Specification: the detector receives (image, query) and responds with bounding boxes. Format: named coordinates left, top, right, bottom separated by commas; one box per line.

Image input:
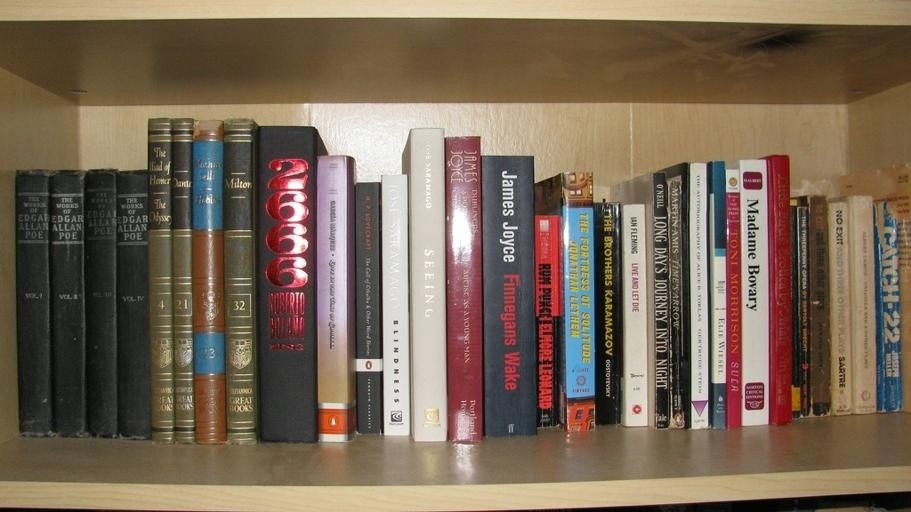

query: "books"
left=16, top=118, right=911, bottom=447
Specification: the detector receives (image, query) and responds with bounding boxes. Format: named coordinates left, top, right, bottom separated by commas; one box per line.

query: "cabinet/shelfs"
left=1, top=1, right=911, bottom=511
